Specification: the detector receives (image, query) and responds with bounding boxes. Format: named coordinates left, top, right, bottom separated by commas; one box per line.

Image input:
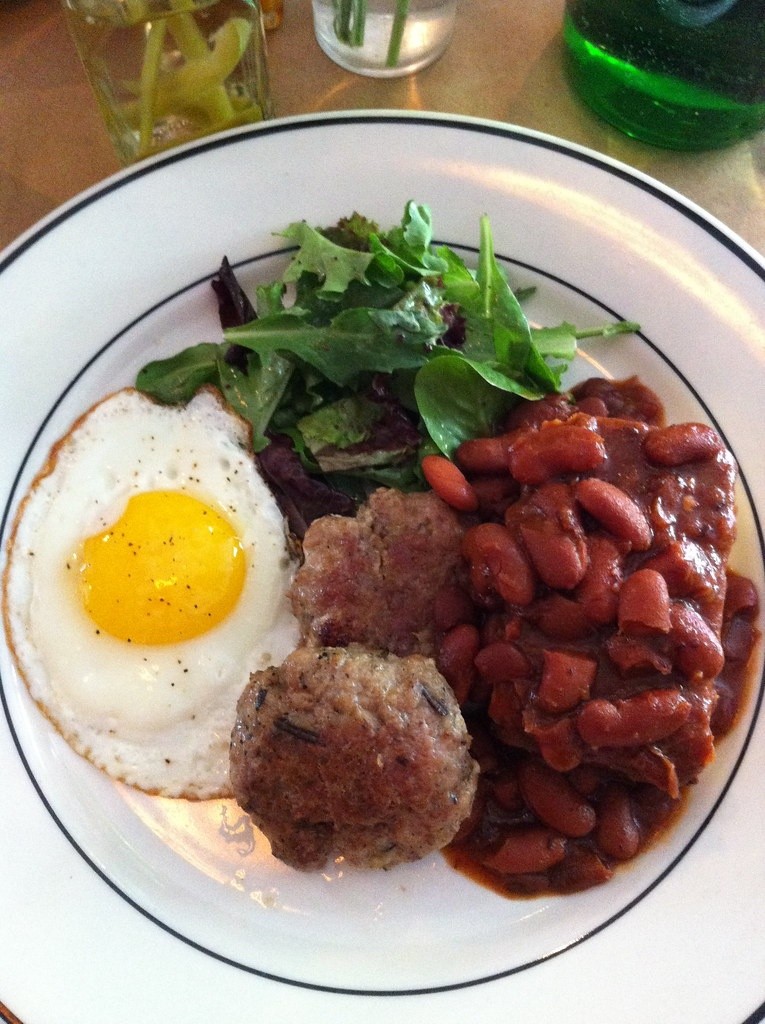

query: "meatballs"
left=226, top=484, right=487, bottom=877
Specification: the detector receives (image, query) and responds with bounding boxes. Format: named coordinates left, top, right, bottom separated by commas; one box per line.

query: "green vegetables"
left=123, top=197, right=646, bottom=536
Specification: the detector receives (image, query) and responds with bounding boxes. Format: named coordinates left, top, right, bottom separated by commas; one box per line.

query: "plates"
left=0, top=108, right=761, bottom=1024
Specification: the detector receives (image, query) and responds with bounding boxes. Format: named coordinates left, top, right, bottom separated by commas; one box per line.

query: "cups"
left=255, top=0, right=285, bottom=33
left=60, top=0, right=273, bottom=172
left=312, top=0, right=459, bottom=79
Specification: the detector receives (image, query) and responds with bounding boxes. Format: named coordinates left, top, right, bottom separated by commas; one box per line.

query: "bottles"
left=558, top=1, right=764, bottom=157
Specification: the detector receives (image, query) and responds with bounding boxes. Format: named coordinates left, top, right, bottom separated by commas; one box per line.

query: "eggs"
left=3, top=383, right=299, bottom=805
